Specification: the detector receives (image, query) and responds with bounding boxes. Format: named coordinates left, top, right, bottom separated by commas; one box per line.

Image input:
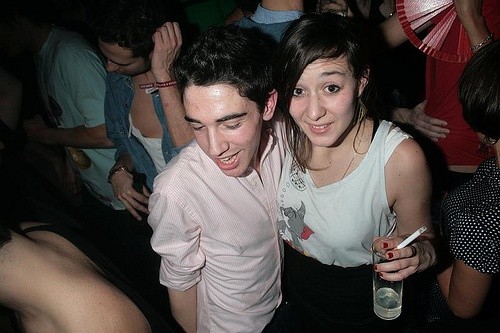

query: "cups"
left=371, top=237, right=404, bottom=320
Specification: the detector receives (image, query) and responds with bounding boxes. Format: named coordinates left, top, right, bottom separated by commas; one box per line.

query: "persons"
left=0, top=0, right=499, bottom=333
